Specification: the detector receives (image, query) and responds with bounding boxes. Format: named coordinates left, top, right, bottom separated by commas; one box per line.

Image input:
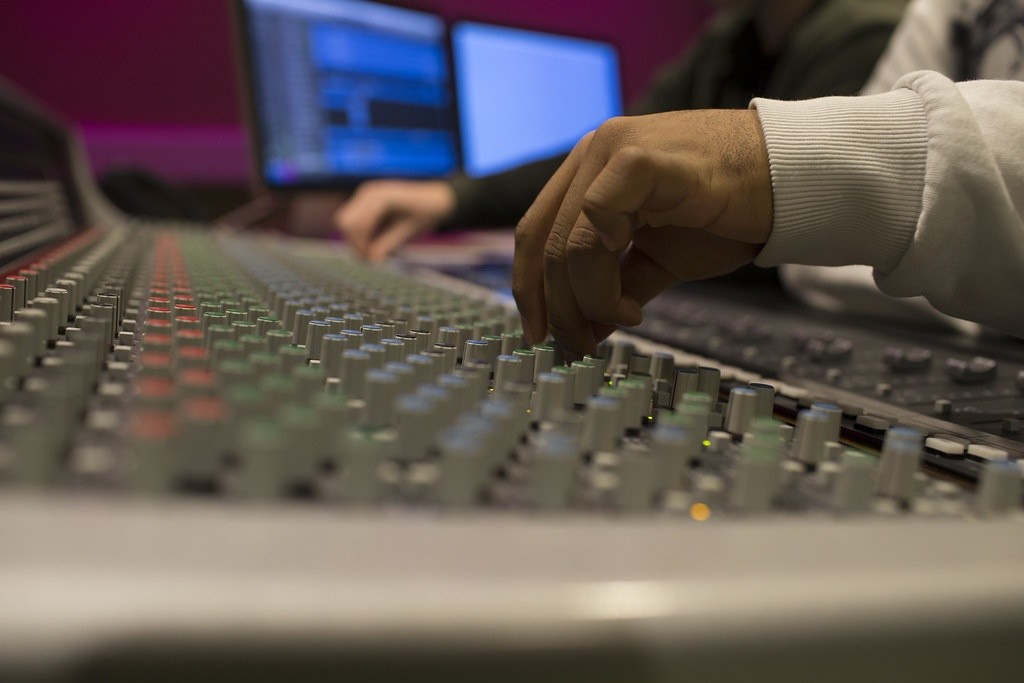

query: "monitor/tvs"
left=445, top=19, right=625, bottom=182
left=229, top=0, right=454, bottom=195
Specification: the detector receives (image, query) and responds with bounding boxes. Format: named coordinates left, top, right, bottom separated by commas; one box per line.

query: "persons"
left=513, top=0, right=1024, bottom=367
left=333, top=0, right=905, bottom=263
